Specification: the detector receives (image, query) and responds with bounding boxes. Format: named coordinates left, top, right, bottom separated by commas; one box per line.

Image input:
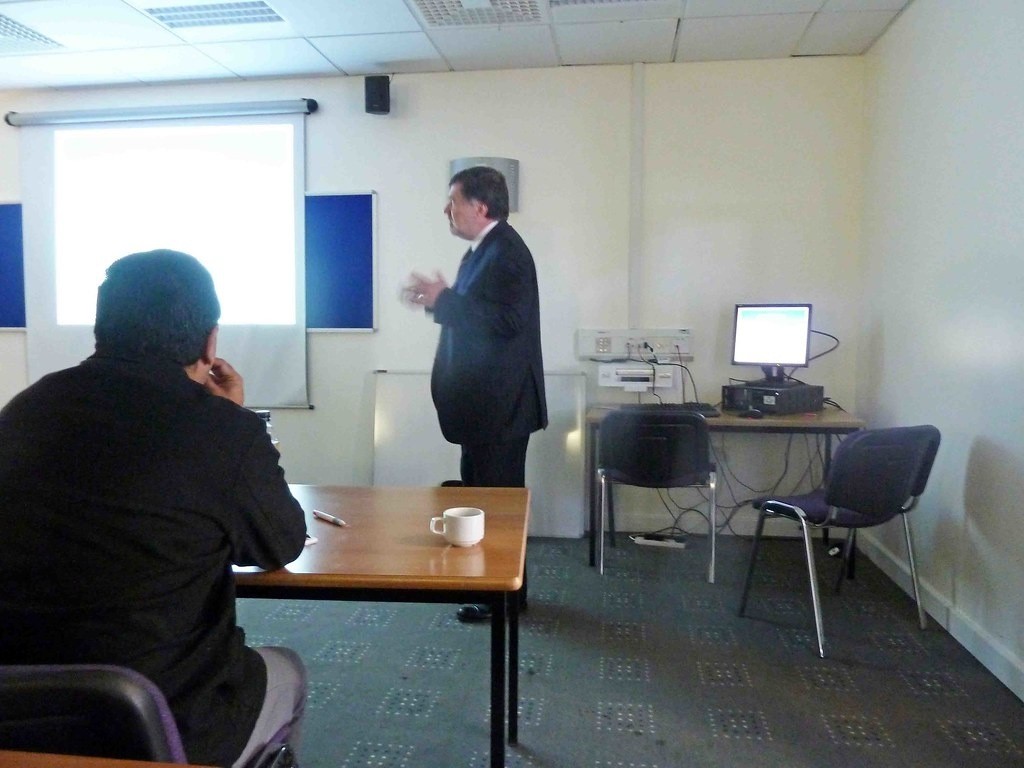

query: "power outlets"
left=627, top=326, right=695, bottom=359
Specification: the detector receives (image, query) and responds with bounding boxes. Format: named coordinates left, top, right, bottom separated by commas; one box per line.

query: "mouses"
left=739, top=409, right=761, bottom=419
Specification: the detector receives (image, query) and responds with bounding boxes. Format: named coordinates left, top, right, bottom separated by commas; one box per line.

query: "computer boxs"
left=721, top=384, right=825, bottom=417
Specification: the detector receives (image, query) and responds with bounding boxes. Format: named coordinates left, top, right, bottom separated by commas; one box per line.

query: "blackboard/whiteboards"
left=0, top=191, right=380, bottom=334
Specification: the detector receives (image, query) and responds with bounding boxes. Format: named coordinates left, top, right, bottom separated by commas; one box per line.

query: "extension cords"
left=635, top=535, right=686, bottom=549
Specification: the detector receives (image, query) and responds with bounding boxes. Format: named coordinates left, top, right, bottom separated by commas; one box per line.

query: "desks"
left=586, top=402, right=866, bottom=580
left=233, top=483, right=532, bottom=768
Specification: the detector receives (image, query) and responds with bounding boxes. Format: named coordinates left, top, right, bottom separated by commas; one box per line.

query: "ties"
left=457, top=246, right=473, bottom=279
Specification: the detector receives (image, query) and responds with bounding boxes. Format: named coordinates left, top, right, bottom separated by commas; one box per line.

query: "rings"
left=416, top=293, right=422, bottom=299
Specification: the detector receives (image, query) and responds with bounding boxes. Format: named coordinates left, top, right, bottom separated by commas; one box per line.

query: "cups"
left=429, top=507, right=486, bottom=547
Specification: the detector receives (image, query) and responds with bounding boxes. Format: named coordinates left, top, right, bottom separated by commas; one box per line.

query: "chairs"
left=0, top=664, right=294, bottom=768
left=595, top=411, right=718, bottom=585
left=737, top=423, right=942, bottom=659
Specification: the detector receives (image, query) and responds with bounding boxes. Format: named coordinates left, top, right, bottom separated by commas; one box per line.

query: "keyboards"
left=620, top=403, right=721, bottom=418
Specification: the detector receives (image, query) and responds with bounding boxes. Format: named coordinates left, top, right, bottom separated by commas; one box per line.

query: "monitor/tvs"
left=732, top=303, right=812, bottom=386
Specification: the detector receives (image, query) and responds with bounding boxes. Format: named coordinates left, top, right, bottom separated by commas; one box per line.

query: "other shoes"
left=503, top=599, right=528, bottom=620
left=457, top=604, right=492, bottom=620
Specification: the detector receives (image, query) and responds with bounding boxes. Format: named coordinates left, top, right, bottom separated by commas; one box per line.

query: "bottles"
left=255, top=408, right=281, bottom=464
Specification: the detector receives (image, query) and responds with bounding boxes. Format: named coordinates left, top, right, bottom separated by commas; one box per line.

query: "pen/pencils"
left=312, top=509, right=346, bottom=527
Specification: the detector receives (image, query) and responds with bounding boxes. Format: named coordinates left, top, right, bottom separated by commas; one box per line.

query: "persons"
left=401, top=167, right=549, bottom=624
left=0, top=249, right=306, bottom=768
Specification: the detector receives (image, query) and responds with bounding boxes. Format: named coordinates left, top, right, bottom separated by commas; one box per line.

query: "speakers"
left=363, top=75, right=390, bottom=114
